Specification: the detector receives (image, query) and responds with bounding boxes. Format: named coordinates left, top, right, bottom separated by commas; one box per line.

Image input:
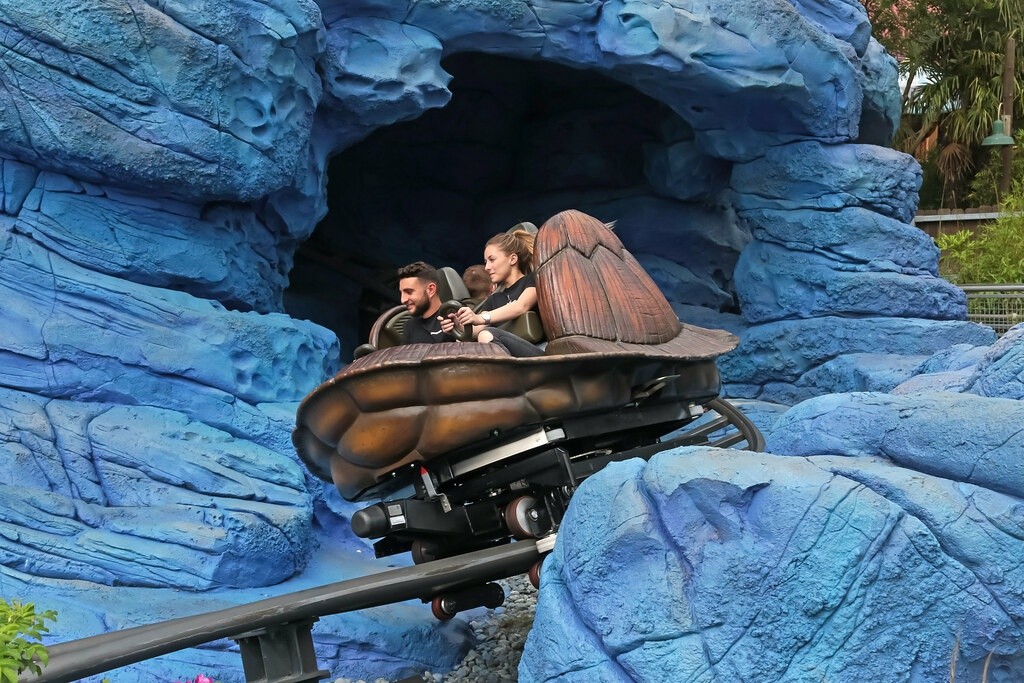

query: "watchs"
left=481, top=310, right=491, bottom=325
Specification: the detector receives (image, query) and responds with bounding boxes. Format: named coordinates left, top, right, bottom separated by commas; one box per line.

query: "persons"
left=396, top=231, right=537, bottom=343
